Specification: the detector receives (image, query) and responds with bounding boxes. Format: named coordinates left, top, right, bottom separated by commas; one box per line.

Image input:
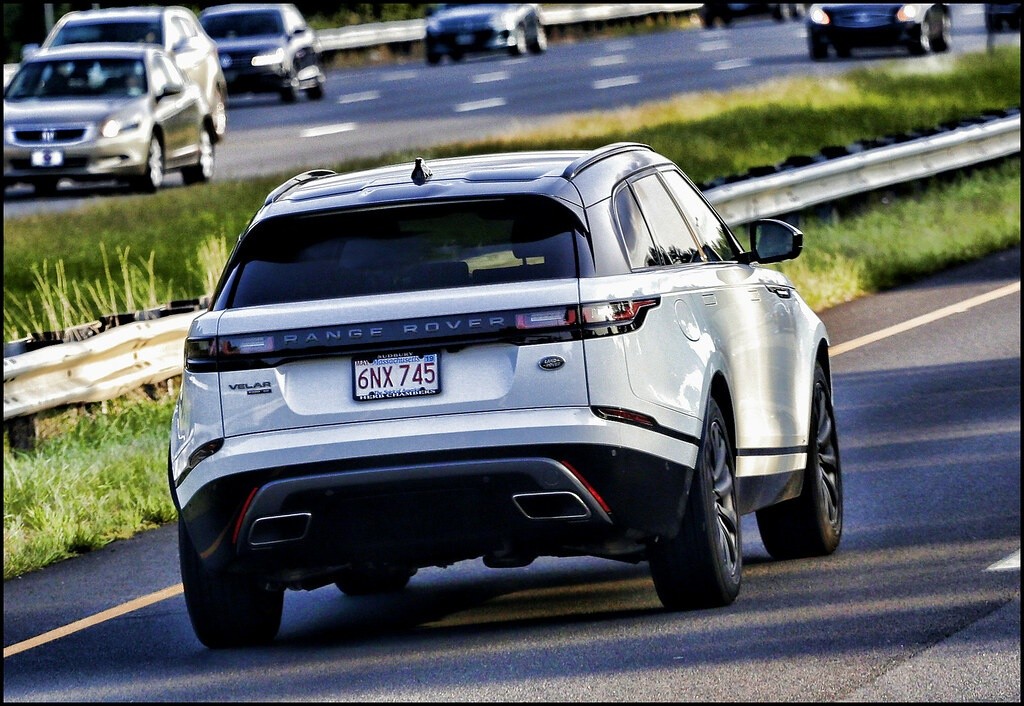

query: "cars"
left=806, top=3, right=954, bottom=57
left=985, top=4, right=1020, bottom=32
left=3, top=42, right=216, bottom=190
left=696, top=4, right=807, bottom=27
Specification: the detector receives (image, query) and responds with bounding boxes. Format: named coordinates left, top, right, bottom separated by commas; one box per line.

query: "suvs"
left=424, top=3, right=548, bottom=63
left=166, top=140, right=844, bottom=647
left=37, top=7, right=229, bottom=142
left=199, top=3, right=326, bottom=103
